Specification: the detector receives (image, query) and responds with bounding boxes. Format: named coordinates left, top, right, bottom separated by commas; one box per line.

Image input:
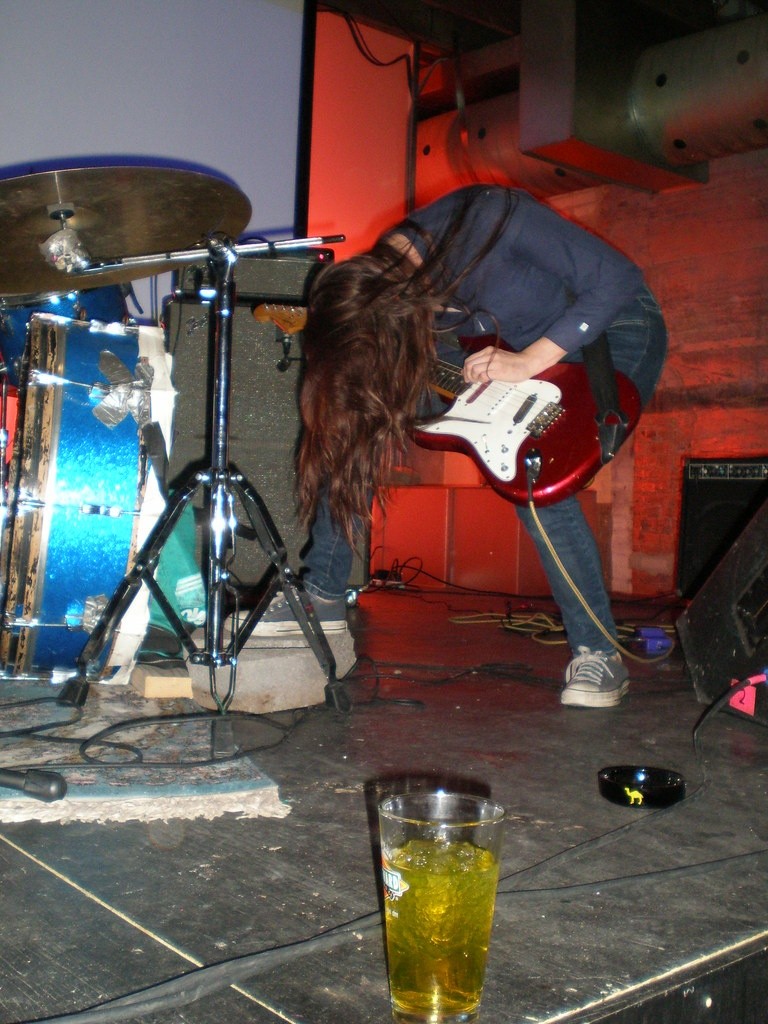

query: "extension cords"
left=371, top=579, right=402, bottom=588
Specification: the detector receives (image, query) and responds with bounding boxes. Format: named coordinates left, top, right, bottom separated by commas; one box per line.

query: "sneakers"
left=224, top=584, right=347, bottom=638
left=560, top=645, right=629, bottom=707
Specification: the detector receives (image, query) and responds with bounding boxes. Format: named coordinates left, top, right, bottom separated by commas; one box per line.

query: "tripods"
left=54, top=260, right=354, bottom=762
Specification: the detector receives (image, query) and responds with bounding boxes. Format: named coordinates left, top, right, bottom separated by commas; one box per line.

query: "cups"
left=376, top=791, right=506, bottom=1024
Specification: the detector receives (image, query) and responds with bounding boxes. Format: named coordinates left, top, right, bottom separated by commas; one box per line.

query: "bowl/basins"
left=598, top=764, right=685, bottom=809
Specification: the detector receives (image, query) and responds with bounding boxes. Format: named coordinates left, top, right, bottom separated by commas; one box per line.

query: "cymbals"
left=0, top=164, right=253, bottom=298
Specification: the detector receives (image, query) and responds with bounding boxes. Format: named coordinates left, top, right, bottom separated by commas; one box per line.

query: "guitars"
left=251, top=298, right=643, bottom=509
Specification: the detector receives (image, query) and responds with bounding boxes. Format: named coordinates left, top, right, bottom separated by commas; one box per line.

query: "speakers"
left=676, top=497, right=768, bottom=725
left=675, top=455, right=767, bottom=609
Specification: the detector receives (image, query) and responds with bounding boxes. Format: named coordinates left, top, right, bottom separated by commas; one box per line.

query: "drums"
left=1, top=311, right=155, bottom=680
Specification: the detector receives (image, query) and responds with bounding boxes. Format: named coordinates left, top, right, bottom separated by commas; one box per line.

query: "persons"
left=225, top=184, right=669, bottom=708
